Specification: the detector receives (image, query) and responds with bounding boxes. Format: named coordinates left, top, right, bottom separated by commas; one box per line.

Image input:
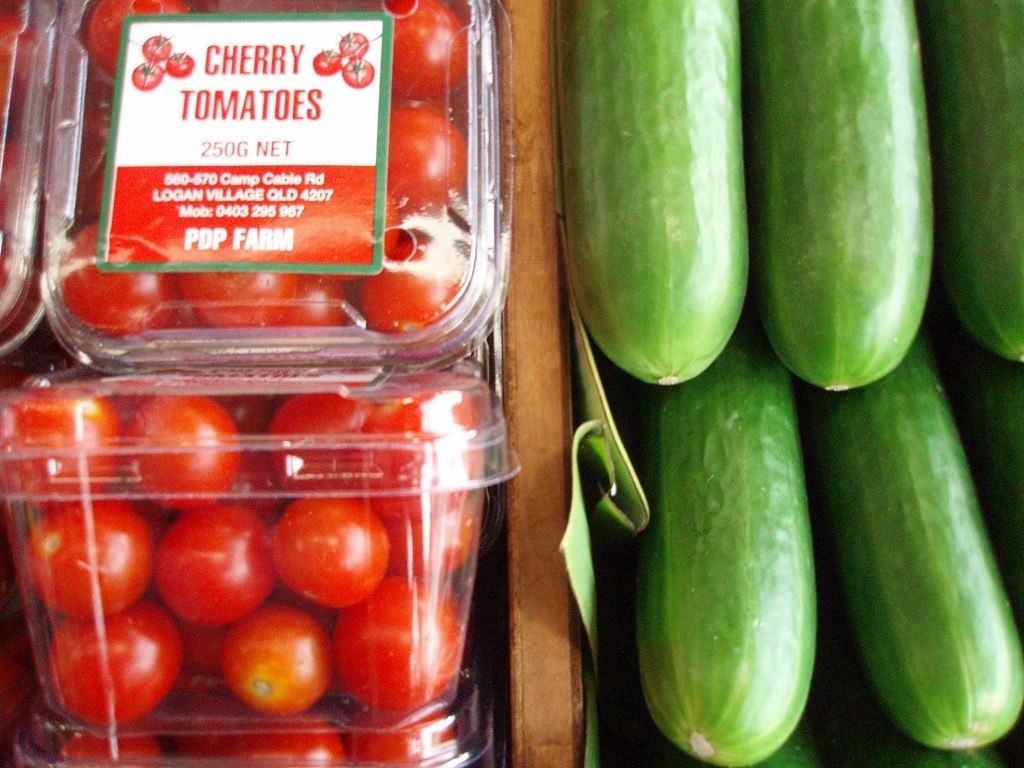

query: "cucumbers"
left=558, top=0, right=1024, bottom=768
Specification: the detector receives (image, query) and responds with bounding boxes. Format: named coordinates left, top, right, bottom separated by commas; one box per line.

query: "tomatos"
left=0, top=0, right=494, bottom=768
